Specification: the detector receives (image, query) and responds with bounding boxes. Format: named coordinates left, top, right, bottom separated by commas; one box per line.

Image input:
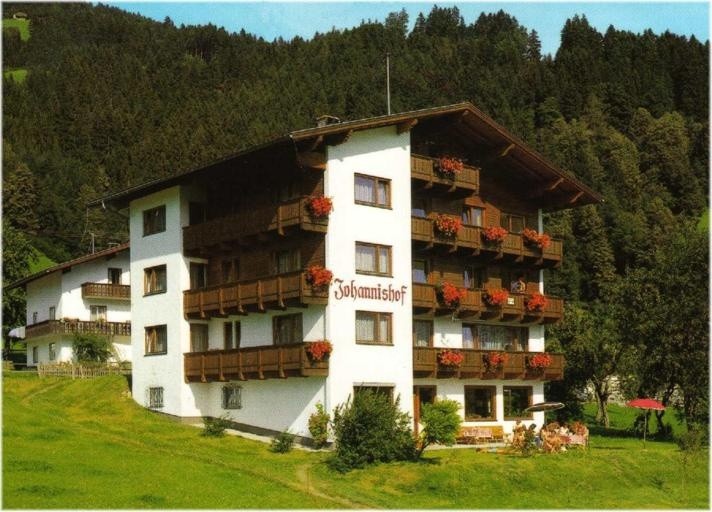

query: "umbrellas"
left=523, top=401, right=565, bottom=425
left=625, top=399, right=666, bottom=448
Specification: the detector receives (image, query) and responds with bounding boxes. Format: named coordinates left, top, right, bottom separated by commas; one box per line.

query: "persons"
left=476, top=448, right=513, bottom=454
left=512, top=277, right=525, bottom=293
left=512, top=419, right=587, bottom=453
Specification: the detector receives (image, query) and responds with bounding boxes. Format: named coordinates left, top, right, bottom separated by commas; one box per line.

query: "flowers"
left=433, top=158, right=553, bottom=375
left=304, top=197, right=332, bottom=367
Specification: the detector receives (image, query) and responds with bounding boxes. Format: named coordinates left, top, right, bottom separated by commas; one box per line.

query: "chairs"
left=456, top=418, right=591, bottom=453
